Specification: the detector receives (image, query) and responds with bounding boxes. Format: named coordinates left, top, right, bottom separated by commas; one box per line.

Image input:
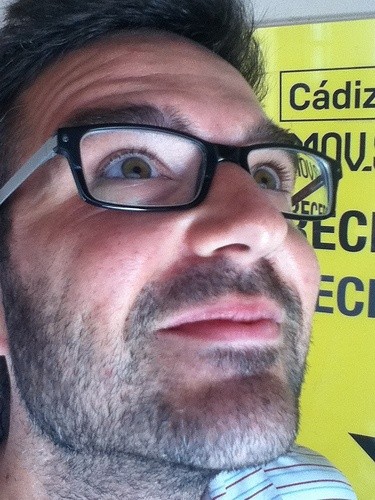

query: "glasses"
left=1, top=122, right=344, bottom=222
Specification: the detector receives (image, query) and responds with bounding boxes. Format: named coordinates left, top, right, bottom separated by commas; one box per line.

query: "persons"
left=0, top=0, right=360, bottom=500
